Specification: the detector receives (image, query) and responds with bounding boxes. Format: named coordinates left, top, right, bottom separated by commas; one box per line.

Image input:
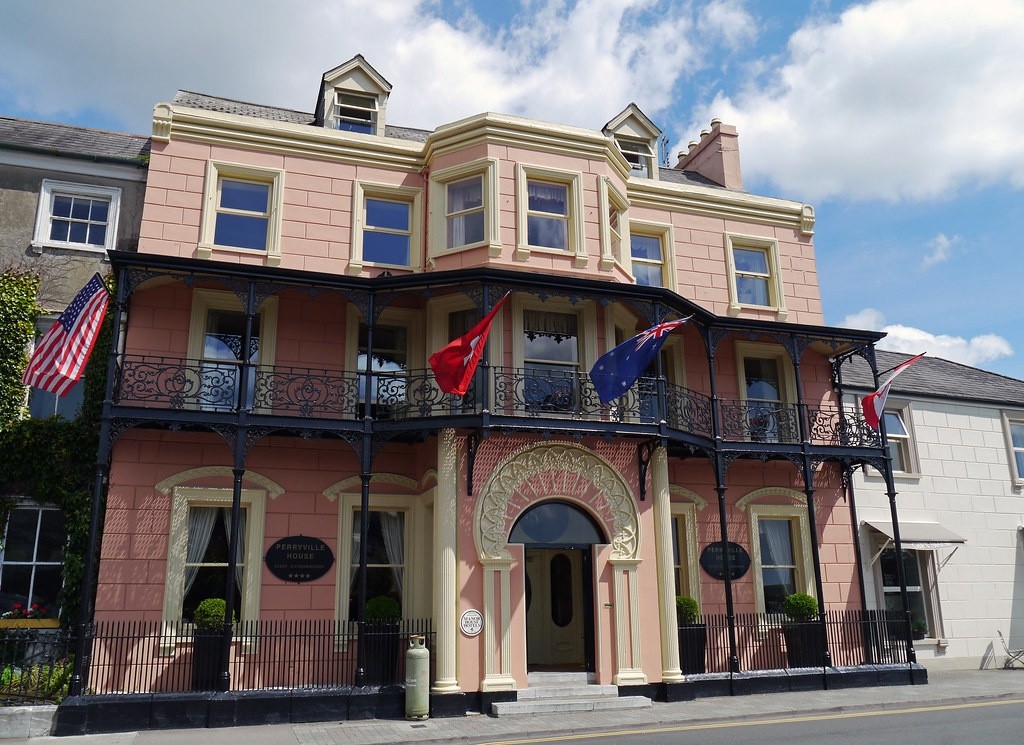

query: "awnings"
left=866, top=522, right=968, bottom=549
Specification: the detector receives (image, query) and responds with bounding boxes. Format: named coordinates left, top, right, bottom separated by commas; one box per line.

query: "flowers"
left=1, top=603, right=49, bottom=619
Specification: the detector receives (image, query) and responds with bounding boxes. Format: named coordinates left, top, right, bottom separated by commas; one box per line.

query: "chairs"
left=997, top=630, right=1024, bottom=669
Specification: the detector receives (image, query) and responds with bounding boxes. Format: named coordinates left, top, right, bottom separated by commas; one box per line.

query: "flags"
left=22, top=272, right=111, bottom=398
left=589, top=313, right=695, bottom=404
left=861, top=352, right=925, bottom=432
left=428, top=289, right=512, bottom=397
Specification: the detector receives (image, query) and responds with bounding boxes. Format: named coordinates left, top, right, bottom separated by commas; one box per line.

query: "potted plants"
left=675, top=597, right=707, bottom=675
left=192, top=598, right=236, bottom=692
left=912, top=619, right=927, bottom=639
left=364, top=597, right=401, bottom=685
left=782, top=592, right=825, bottom=668
